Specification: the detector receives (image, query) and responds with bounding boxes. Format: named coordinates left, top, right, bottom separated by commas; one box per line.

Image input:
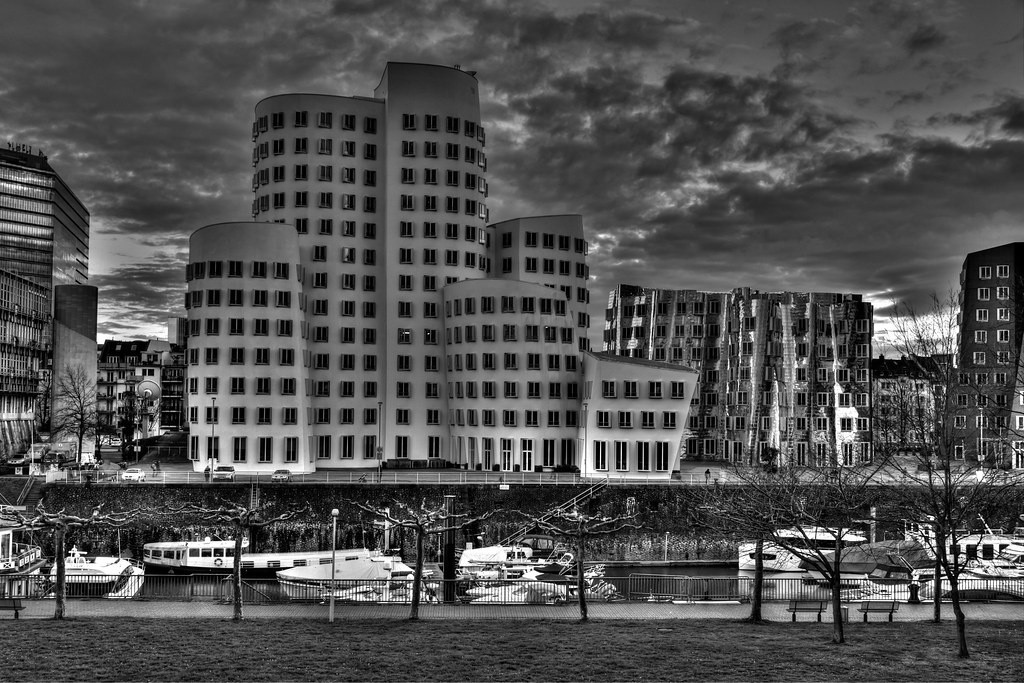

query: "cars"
left=271, top=469, right=294, bottom=482
left=7, top=453, right=32, bottom=467
left=121, top=468, right=146, bottom=482
left=27, top=442, right=99, bottom=472
left=111, top=438, right=122, bottom=447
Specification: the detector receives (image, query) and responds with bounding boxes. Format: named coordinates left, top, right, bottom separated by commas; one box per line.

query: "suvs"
left=213, top=465, right=236, bottom=483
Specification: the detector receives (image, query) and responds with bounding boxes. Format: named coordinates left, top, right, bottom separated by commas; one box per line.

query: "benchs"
left=0, top=598, right=27, bottom=620
left=857, top=601, right=900, bottom=623
left=787, top=599, right=827, bottom=622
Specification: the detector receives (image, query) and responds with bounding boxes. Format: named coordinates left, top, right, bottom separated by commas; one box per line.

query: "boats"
left=0, top=527, right=46, bottom=599
left=43, top=545, right=147, bottom=602
left=139, top=539, right=621, bottom=606
left=737, top=525, right=1023, bottom=604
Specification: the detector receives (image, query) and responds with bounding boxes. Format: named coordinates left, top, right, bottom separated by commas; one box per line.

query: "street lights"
left=583, top=402, right=591, bottom=482
left=377, top=401, right=383, bottom=483
left=211, top=396, right=215, bottom=482
left=328, top=508, right=340, bottom=622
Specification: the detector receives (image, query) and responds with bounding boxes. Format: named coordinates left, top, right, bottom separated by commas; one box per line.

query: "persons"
left=705, top=469, right=711, bottom=484
left=204, top=466, right=210, bottom=482
left=150, top=459, right=160, bottom=478
left=377, top=465, right=383, bottom=483
